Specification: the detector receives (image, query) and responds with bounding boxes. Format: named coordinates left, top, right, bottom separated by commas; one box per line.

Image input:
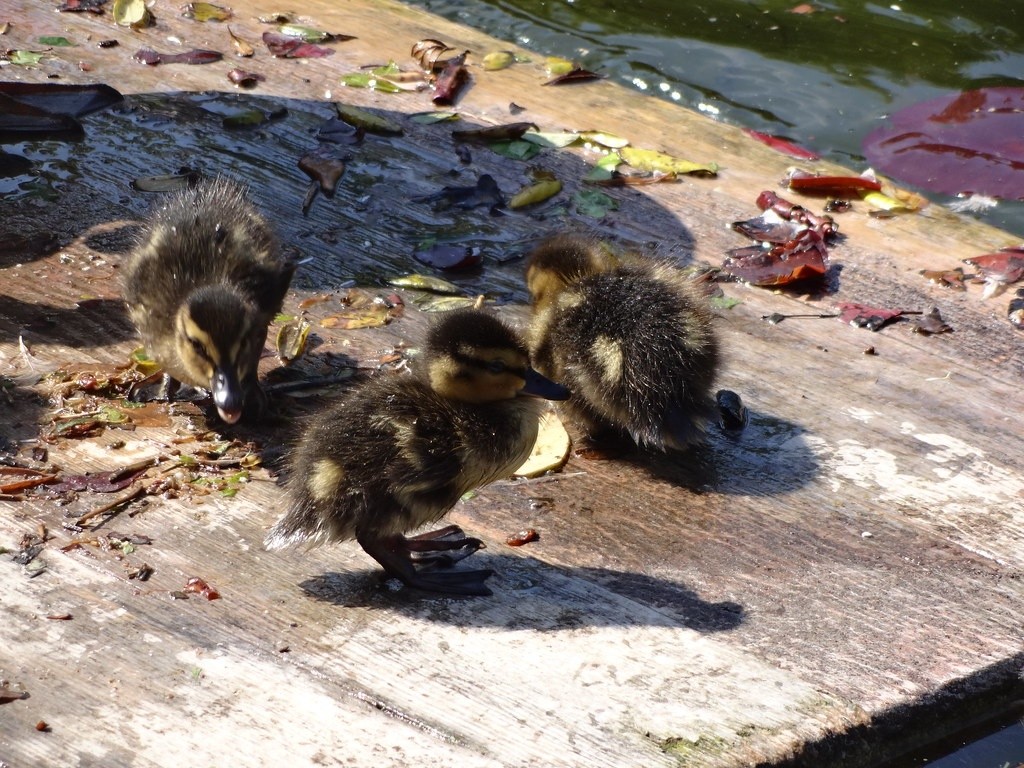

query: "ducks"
left=519, top=233, right=721, bottom=441
left=113, top=173, right=296, bottom=425
left=259, top=306, right=574, bottom=598
